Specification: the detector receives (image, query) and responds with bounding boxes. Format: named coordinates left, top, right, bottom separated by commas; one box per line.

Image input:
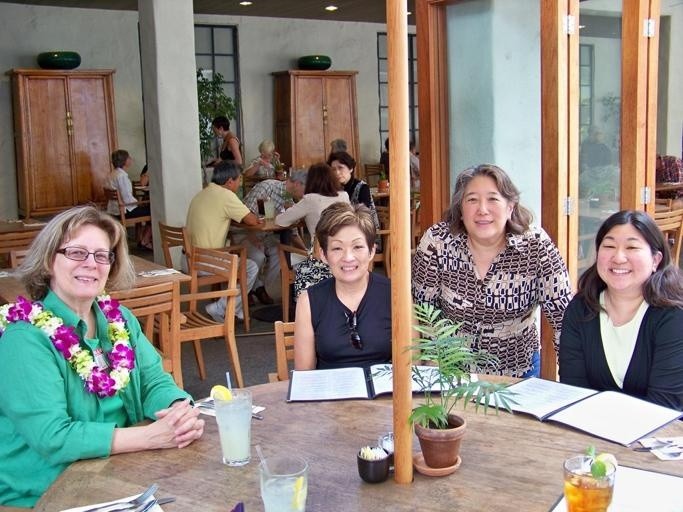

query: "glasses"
left=344, top=311, right=363, bottom=351
left=56, top=247, right=116, bottom=265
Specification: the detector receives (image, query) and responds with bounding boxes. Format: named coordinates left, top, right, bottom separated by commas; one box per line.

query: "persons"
left=293, top=202, right=421, bottom=372
left=1, top=206, right=205, bottom=507
left=139, top=162, right=150, bottom=186
left=231, top=168, right=308, bottom=305
left=581, top=127, right=611, bottom=176
left=411, top=163, right=572, bottom=378
left=243, top=139, right=279, bottom=179
left=327, top=151, right=384, bottom=253
left=330, top=140, right=347, bottom=152
left=106, top=150, right=153, bottom=250
left=381, top=138, right=421, bottom=196
left=560, top=209, right=683, bottom=412
left=208, top=116, right=242, bottom=166
left=275, top=163, right=354, bottom=302
left=184, top=160, right=259, bottom=323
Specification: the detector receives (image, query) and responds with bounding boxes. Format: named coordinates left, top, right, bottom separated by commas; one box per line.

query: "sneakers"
left=206, top=301, right=225, bottom=323
left=235, top=307, right=251, bottom=322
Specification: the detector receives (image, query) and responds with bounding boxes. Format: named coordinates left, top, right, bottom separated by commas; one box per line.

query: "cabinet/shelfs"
left=5, top=68, right=118, bottom=222
left=270, top=70, right=362, bottom=180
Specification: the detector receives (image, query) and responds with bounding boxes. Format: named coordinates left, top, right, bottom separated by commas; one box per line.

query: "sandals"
left=137, top=240, right=153, bottom=253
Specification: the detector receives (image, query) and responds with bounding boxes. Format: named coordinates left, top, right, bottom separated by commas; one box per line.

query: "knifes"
left=109, top=499, right=174, bottom=512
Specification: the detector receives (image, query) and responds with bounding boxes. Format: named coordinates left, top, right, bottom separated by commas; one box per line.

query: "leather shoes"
left=248, top=291, right=255, bottom=307
left=255, top=286, right=274, bottom=305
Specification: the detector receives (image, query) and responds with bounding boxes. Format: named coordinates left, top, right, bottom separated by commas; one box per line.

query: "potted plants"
left=370, top=301, right=524, bottom=468
left=357, top=445, right=391, bottom=484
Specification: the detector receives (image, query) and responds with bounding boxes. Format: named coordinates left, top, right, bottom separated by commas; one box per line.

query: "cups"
left=214, top=389, right=253, bottom=467
left=564, top=455, right=616, bottom=512
left=358, top=446, right=388, bottom=485
left=260, top=454, right=307, bottom=511
left=382, top=435, right=394, bottom=471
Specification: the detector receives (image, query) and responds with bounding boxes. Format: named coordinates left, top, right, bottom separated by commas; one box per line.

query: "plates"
left=413, top=451, right=460, bottom=477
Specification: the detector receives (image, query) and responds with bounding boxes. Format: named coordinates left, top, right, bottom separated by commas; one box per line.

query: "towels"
left=23, top=222, right=48, bottom=226
left=1, top=271, right=16, bottom=277
left=138, top=268, right=180, bottom=277
left=639, top=438, right=683, bottom=462
left=192, top=395, right=266, bottom=419
left=57, top=492, right=167, bottom=512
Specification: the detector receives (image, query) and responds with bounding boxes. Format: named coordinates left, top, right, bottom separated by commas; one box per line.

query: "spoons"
left=134, top=500, right=154, bottom=512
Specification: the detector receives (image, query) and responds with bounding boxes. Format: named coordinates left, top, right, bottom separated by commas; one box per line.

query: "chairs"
left=135, top=248, right=243, bottom=390
left=0, top=233, right=42, bottom=255
left=132, top=181, right=147, bottom=201
left=11, top=249, right=28, bottom=268
left=655, top=180, right=676, bottom=193
left=103, top=187, right=151, bottom=229
left=367, top=205, right=391, bottom=280
left=365, top=164, right=384, bottom=185
left=652, top=209, right=679, bottom=269
left=653, top=199, right=671, bottom=243
left=277, top=236, right=322, bottom=323
left=109, top=280, right=182, bottom=391
left=157, top=222, right=250, bottom=333
left=655, top=209, right=683, bottom=271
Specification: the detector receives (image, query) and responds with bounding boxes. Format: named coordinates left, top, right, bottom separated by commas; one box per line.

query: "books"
left=473, top=378, right=683, bottom=446
left=285, top=364, right=456, bottom=402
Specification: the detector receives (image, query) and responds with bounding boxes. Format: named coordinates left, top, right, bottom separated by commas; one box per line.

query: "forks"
left=198, top=401, right=264, bottom=421
left=83, top=483, right=158, bottom=512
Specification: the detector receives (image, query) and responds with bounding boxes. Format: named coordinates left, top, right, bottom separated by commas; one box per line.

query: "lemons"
left=590, top=454, right=616, bottom=477
left=292, top=476, right=305, bottom=512
left=209, top=384, right=232, bottom=404
